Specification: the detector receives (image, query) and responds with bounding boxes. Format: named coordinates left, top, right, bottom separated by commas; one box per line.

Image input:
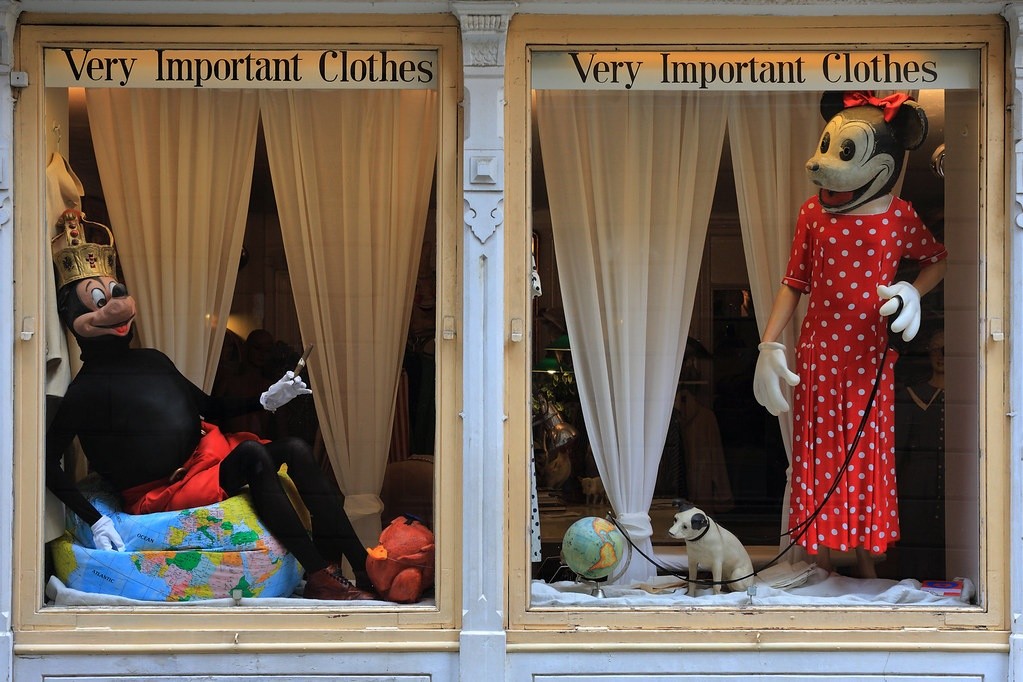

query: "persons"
left=753, top=89, right=949, bottom=578
left=44, top=208, right=386, bottom=602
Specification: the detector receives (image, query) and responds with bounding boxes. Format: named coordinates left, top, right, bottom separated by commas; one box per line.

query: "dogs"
left=668, top=499, right=754, bottom=597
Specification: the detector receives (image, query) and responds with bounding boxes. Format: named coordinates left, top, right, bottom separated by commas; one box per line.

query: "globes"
left=560, top=511, right=633, bottom=598
